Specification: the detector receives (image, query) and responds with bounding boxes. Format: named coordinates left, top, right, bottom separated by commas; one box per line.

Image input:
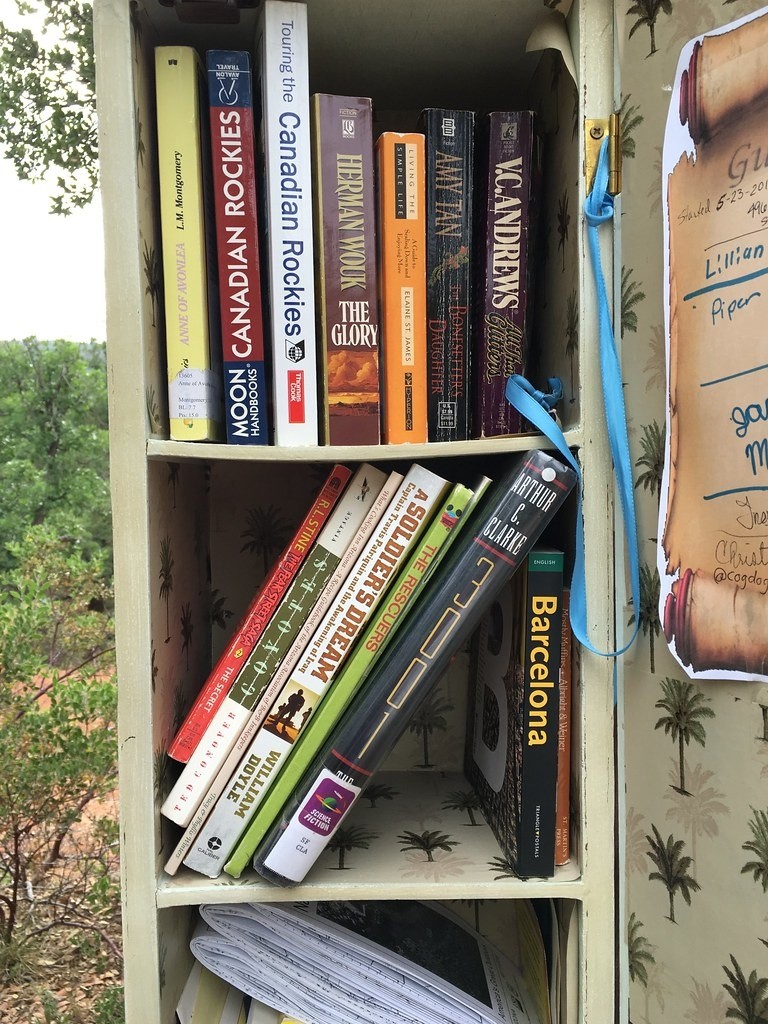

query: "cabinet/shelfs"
left=92, top=0, right=618, bottom=1024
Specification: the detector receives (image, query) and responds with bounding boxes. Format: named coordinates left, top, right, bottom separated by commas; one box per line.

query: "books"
left=153, top=0, right=533, bottom=447
left=158, top=448, right=579, bottom=890
